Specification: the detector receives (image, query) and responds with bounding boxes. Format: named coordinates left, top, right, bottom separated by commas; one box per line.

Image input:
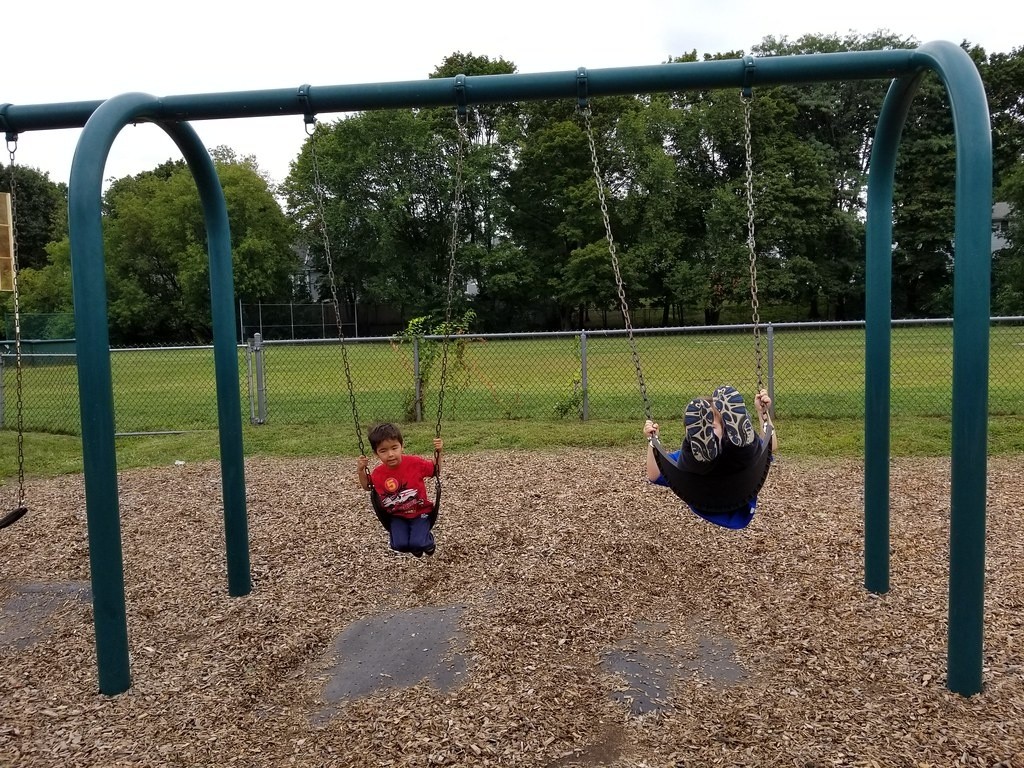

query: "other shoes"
left=424, top=532, right=435, bottom=556
left=712, top=385, right=754, bottom=447
left=684, top=399, right=718, bottom=462
left=411, top=551, right=423, bottom=557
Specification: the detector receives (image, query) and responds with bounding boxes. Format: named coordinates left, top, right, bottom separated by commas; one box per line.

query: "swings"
left=0, top=129, right=30, bottom=527
left=581, top=89, right=776, bottom=510
left=303, top=112, right=463, bottom=529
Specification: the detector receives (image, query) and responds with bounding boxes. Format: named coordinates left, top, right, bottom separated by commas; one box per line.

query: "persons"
left=643, top=384, right=777, bottom=529
left=356, top=422, right=443, bottom=558
